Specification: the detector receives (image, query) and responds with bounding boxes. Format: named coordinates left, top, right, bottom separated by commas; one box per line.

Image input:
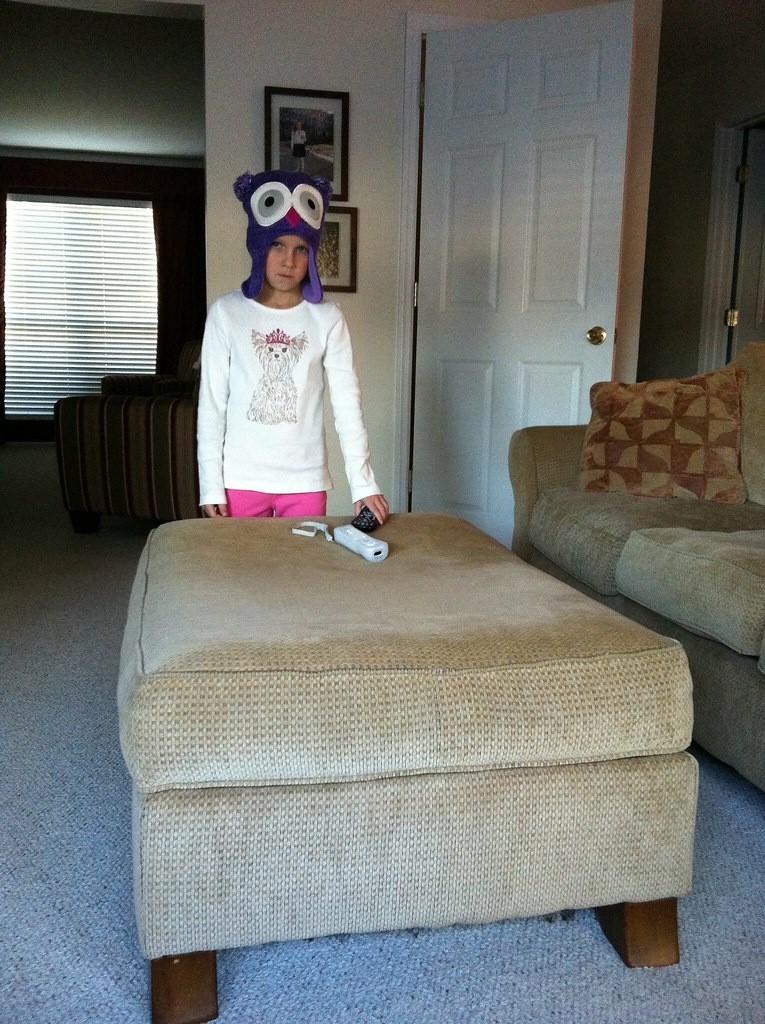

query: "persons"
left=290, top=118, right=308, bottom=173
left=195, top=166, right=390, bottom=527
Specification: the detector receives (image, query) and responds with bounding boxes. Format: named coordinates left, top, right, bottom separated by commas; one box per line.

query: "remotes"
left=351, top=505, right=379, bottom=533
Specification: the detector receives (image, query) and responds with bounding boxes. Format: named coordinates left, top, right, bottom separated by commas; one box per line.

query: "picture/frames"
left=311, top=205, right=359, bottom=294
left=262, top=87, right=349, bottom=201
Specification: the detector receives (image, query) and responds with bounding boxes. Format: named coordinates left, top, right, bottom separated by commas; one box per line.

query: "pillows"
left=577, top=365, right=751, bottom=511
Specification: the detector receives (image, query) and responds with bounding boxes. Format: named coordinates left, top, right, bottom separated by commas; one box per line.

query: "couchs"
left=507, top=339, right=764, bottom=795
left=102, top=330, right=203, bottom=403
left=51, top=384, right=203, bottom=531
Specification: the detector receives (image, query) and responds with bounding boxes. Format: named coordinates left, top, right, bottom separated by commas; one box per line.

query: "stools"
left=115, top=509, right=701, bottom=1024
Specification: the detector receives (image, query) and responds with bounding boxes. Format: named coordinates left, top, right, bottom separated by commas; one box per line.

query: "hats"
left=233, top=170, right=332, bottom=304
